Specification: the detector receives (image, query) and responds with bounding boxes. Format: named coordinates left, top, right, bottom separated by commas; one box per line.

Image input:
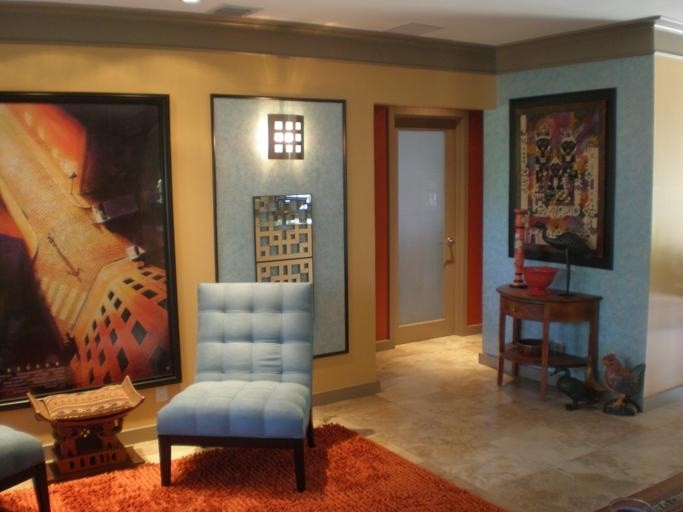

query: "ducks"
left=551, top=365, right=592, bottom=410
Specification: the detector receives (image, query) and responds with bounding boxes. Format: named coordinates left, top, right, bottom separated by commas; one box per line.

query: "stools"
left=26, top=374, right=145, bottom=486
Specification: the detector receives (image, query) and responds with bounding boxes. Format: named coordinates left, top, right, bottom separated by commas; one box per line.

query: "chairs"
left=0, top=425, right=51, bottom=511
left=155, top=282, right=316, bottom=493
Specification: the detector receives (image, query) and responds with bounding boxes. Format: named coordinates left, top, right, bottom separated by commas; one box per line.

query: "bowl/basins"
left=521, top=265, right=559, bottom=297
left=516, top=339, right=549, bottom=358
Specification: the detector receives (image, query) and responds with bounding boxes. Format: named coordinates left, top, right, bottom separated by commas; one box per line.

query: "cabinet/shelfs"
left=496, top=283, right=603, bottom=402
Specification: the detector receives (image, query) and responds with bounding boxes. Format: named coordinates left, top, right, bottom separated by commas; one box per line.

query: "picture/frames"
left=509, top=87, right=616, bottom=270
left=0, top=91, right=182, bottom=413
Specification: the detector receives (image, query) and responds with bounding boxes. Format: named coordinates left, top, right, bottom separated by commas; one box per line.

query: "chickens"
left=601, top=353, right=646, bottom=409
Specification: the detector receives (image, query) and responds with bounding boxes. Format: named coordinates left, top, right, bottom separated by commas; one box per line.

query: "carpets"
left=0, top=422, right=508, bottom=512
left=593, top=472, right=683, bottom=511
